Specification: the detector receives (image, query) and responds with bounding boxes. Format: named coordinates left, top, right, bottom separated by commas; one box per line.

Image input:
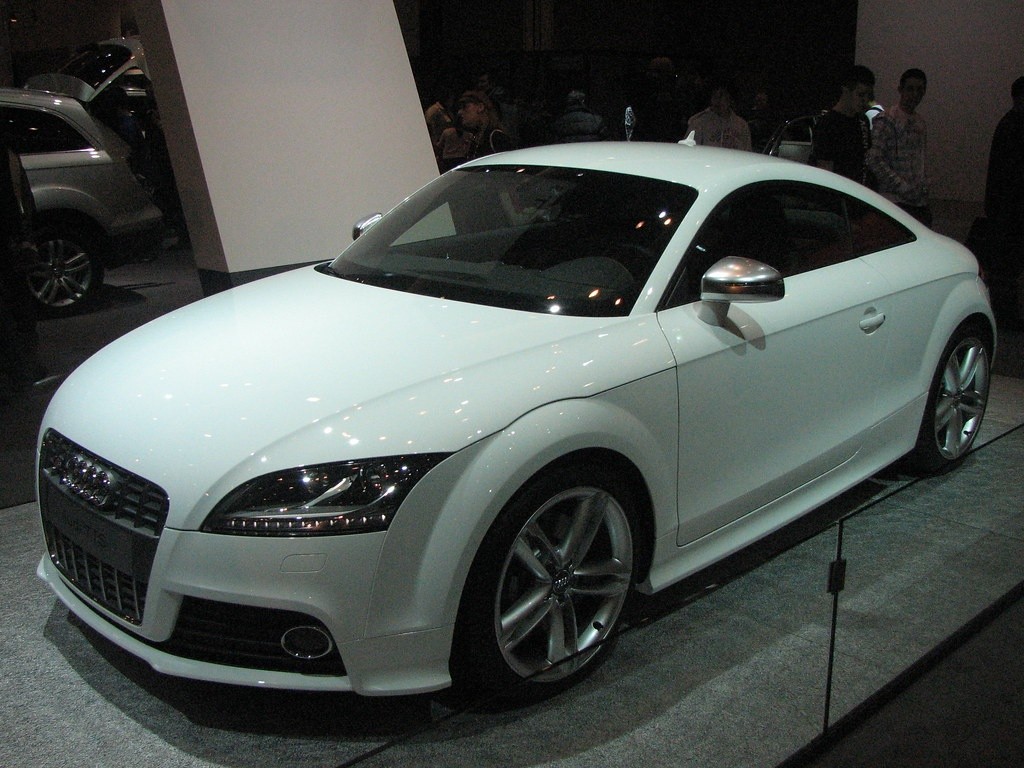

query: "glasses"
left=858, top=92, right=874, bottom=102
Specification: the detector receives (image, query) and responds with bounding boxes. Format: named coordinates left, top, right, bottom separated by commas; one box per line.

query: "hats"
left=459, top=90, right=492, bottom=111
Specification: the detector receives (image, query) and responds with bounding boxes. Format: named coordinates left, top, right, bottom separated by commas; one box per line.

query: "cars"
left=31, top=35, right=182, bottom=211
left=1, top=88, right=164, bottom=322
left=36, top=144, right=997, bottom=728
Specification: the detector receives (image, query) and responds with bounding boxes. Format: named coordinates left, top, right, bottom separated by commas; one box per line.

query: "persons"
left=419, top=62, right=1023, bottom=329
